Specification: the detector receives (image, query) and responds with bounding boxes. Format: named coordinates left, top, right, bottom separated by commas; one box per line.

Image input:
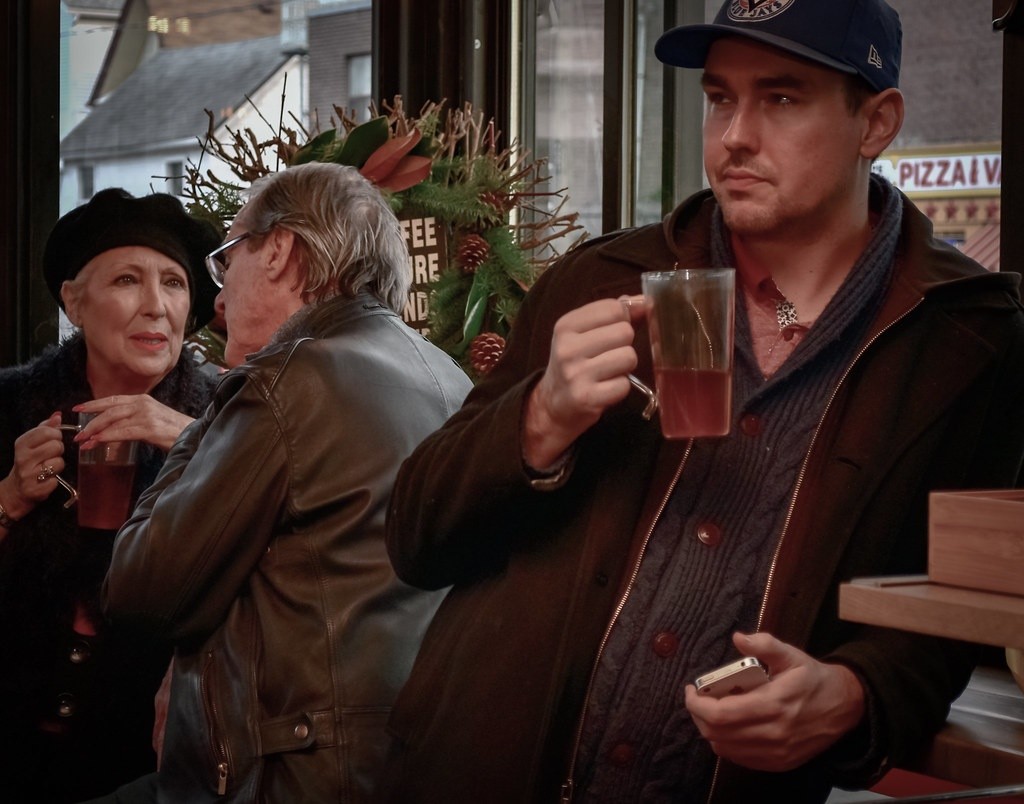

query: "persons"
left=76, top=159, right=485, bottom=804
left=382, top=0, right=1023, bottom=804
left=0, top=188, right=242, bottom=804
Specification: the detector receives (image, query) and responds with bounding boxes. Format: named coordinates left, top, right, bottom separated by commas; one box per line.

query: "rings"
left=37, top=465, right=54, bottom=482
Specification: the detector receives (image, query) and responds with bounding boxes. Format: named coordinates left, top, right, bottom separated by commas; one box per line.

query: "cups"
left=616, top=270, right=737, bottom=438
left=53, top=412, right=141, bottom=529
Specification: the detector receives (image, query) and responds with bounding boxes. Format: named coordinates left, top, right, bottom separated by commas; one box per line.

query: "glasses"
left=204, top=225, right=277, bottom=287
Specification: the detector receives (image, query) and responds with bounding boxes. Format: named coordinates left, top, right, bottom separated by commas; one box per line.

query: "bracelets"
left=0, top=506, right=18, bottom=532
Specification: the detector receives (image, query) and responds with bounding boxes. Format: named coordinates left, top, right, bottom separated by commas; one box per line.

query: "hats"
left=654, top=1, right=903, bottom=93
left=40, top=187, right=227, bottom=338
